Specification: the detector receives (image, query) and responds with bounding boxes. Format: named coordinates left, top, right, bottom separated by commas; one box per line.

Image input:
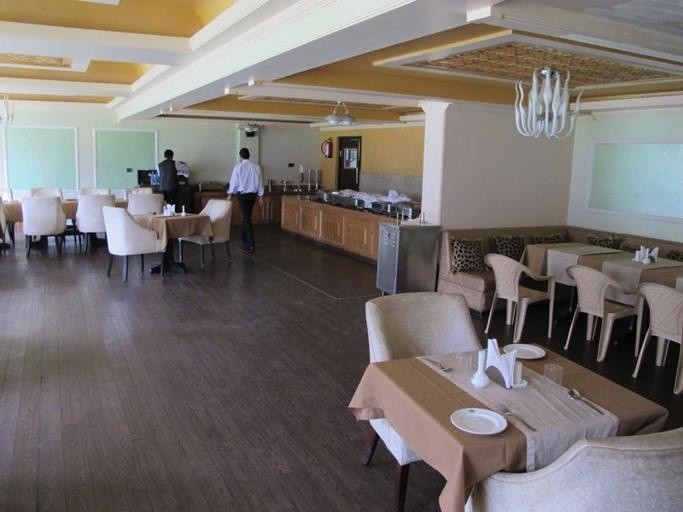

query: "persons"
left=156, top=149, right=190, bottom=211
left=226, top=147, right=265, bottom=251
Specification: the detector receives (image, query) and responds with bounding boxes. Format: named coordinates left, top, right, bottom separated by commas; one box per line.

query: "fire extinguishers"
left=321, top=137, right=333, bottom=158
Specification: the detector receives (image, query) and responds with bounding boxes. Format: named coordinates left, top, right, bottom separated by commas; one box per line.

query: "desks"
left=349, top=343, right=668, bottom=511
left=600, top=252, right=683, bottom=332
left=521, top=244, right=623, bottom=314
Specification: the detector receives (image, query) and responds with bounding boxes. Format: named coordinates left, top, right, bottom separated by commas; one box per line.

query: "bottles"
left=469, top=349, right=490, bottom=388
left=149, top=171, right=159, bottom=187
left=160, top=200, right=186, bottom=217
left=633, top=245, right=651, bottom=265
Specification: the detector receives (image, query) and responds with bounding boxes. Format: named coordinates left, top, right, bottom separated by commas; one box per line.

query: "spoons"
left=566, top=387, right=604, bottom=416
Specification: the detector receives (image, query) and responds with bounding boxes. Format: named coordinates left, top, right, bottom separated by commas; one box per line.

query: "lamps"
left=326, top=101, right=355, bottom=127
left=238, top=120, right=266, bottom=135
left=514, top=65, right=587, bottom=143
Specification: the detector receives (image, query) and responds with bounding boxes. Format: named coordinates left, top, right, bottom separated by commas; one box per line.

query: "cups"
left=543, top=362, right=562, bottom=395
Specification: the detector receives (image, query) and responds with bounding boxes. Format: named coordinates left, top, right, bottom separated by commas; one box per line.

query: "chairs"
left=633, top=277, right=682, bottom=397
left=2, top=185, right=234, bottom=283
left=482, top=253, right=556, bottom=342
left=362, top=291, right=486, bottom=508
left=463, top=424, right=682, bottom=512
left=562, top=263, right=641, bottom=362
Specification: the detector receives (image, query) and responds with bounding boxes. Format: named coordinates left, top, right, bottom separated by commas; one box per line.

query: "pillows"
left=452, top=231, right=627, bottom=275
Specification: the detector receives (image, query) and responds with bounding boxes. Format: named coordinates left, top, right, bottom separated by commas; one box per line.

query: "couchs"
left=436, top=224, right=683, bottom=322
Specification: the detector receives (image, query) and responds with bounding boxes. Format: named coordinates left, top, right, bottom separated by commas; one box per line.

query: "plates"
left=449, top=407, right=508, bottom=436
left=501, top=342, right=546, bottom=361
